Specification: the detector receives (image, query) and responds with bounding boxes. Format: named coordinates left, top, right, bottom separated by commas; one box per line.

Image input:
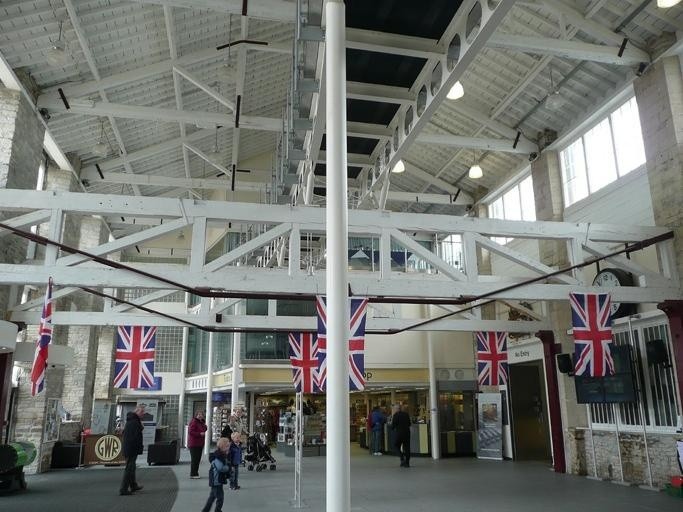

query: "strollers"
left=243, top=431, right=276, bottom=473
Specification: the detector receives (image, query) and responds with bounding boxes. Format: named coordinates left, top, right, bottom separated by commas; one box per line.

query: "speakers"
left=646, top=339, right=668, bottom=365
left=557, top=355, right=572, bottom=373
left=215, top=313, right=222, bottom=323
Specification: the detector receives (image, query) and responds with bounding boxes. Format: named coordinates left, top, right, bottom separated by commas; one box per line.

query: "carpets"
left=0, top=467, right=179, bottom=512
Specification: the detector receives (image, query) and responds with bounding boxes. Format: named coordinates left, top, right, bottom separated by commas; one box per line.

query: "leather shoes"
left=131, top=486, right=143, bottom=492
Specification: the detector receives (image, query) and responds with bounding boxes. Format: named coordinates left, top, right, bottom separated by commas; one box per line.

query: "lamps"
left=446, top=59, right=464, bottom=100
left=46, top=8, right=68, bottom=66
left=92, top=116, right=109, bottom=159
left=469, top=149, right=484, bottom=178
left=220, top=13, right=237, bottom=80
left=546, top=61, right=567, bottom=110
left=207, top=84, right=222, bottom=164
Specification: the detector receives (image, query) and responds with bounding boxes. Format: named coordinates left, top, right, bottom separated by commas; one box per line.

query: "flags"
left=476, top=330, right=510, bottom=386
left=288, top=331, right=318, bottom=393
left=30, top=276, right=53, bottom=397
left=112, top=325, right=156, bottom=389
left=569, top=292, right=617, bottom=377
left=316, top=294, right=366, bottom=393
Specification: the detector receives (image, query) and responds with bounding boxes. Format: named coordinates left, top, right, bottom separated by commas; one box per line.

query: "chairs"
left=147, top=438, right=181, bottom=466
left=51, top=441, right=86, bottom=467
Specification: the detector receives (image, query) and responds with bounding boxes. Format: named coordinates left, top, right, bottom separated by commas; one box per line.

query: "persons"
left=119, top=403, right=145, bottom=496
left=366, top=405, right=411, bottom=469
left=188, top=410, right=207, bottom=479
left=202, top=406, right=251, bottom=512
left=289, top=399, right=315, bottom=415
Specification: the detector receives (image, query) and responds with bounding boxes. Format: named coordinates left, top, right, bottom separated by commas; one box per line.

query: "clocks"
left=591, top=267, right=636, bottom=318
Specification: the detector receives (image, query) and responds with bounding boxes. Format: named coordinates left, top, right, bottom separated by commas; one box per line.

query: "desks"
left=82, top=435, right=125, bottom=464
